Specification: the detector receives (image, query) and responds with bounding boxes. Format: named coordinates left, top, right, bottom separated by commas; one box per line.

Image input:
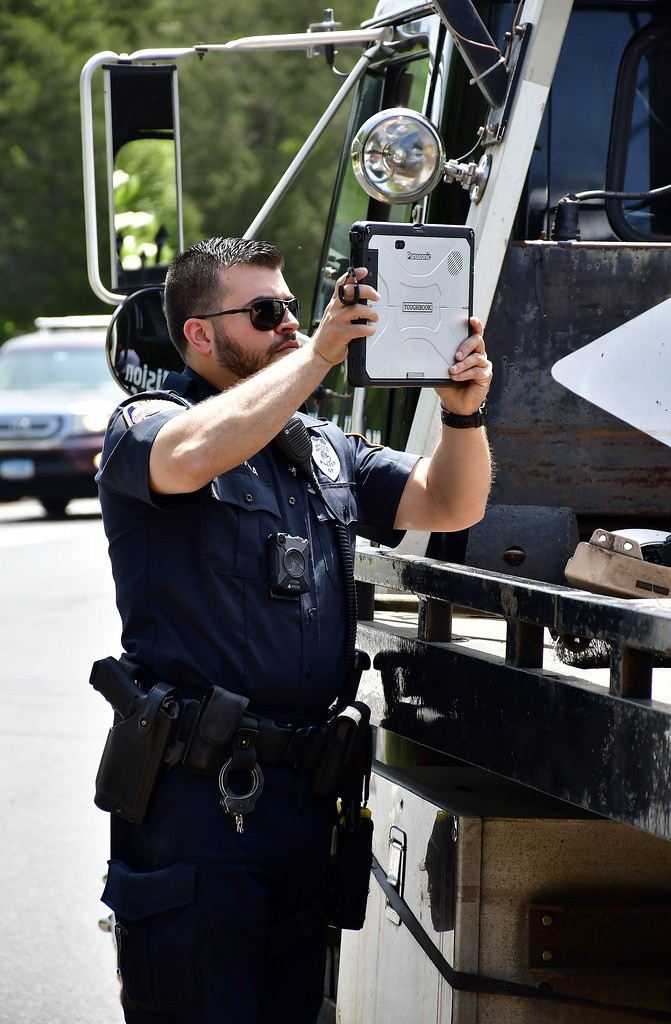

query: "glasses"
left=190, top=296, right=300, bottom=331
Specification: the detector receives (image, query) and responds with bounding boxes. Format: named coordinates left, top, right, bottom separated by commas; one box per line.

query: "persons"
left=95, top=239, right=491, bottom=1024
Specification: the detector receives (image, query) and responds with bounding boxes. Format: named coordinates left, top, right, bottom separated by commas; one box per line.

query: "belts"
left=247, top=714, right=330, bottom=767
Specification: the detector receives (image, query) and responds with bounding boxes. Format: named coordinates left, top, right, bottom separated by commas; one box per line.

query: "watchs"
left=441, top=398, right=488, bottom=428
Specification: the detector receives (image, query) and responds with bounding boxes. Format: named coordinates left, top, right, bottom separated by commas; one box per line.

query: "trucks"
left=81, top=0, right=667, bottom=1024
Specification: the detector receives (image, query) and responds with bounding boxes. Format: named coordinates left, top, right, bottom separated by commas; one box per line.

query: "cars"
left=3, top=314, right=132, bottom=516
